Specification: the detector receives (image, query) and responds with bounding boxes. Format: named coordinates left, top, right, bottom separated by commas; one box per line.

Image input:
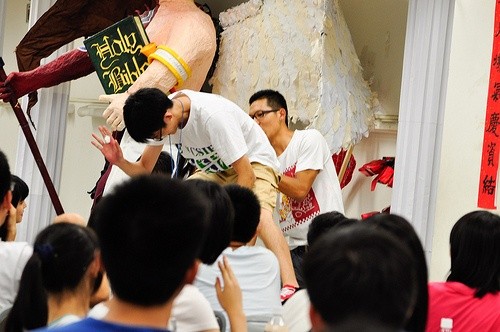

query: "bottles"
left=437, top=318, right=454, bottom=332
left=270, top=310, right=285, bottom=327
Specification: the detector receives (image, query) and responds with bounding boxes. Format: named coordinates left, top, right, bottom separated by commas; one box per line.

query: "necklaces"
left=168, top=97, right=184, bottom=180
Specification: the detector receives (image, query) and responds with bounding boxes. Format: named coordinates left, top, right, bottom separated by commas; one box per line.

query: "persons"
left=0, top=0, right=217, bottom=200
left=0, top=149, right=500, bottom=332
left=90, top=86, right=301, bottom=302
left=247, top=89, right=345, bottom=306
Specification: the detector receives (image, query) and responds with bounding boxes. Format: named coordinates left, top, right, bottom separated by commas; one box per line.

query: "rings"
left=104, top=136, right=111, bottom=143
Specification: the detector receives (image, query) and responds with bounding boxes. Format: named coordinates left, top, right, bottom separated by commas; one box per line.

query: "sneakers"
left=280, top=285, right=301, bottom=304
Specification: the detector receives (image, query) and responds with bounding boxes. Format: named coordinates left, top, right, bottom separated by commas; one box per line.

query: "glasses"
left=153, top=129, right=164, bottom=141
left=248, top=109, right=280, bottom=120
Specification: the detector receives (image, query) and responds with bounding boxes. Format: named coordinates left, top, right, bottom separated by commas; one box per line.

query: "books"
left=82, top=15, right=155, bottom=103
